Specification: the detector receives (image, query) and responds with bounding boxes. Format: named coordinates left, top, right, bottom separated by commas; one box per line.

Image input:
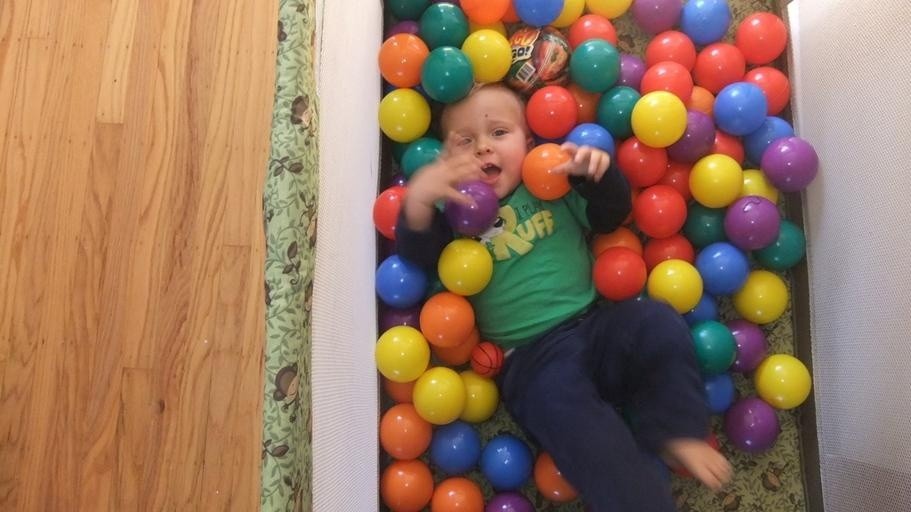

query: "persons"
left=395, top=80, right=732, bottom=511
